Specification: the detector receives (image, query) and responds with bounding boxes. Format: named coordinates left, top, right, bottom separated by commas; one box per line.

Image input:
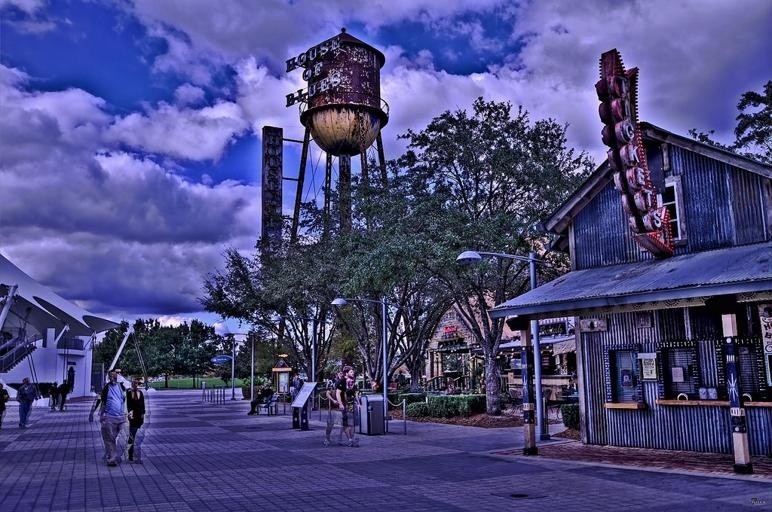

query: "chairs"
left=257, top=394, right=279, bottom=417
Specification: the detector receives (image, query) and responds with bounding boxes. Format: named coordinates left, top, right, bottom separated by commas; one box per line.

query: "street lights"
left=273, top=314, right=319, bottom=412
left=332, top=297, right=390, bottom=433
left=458, top=249, right=547, bottom=442
left=225, top=331, right=258, bottom=417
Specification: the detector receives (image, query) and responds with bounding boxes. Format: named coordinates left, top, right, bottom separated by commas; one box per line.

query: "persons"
left=567, top=379, right=576, bottom=395
left=476, top=362, right=484, bottom=376
left=246, top=366, right=411, bottom=447
left=445, top=377, right=455, bottom=395
left=0, top=378, right=71, bottom=429
left=88, top=367, right=147, bottom=466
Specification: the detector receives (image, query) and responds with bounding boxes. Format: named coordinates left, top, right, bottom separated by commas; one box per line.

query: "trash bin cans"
left=353, top=394, right=384, bottom=435
